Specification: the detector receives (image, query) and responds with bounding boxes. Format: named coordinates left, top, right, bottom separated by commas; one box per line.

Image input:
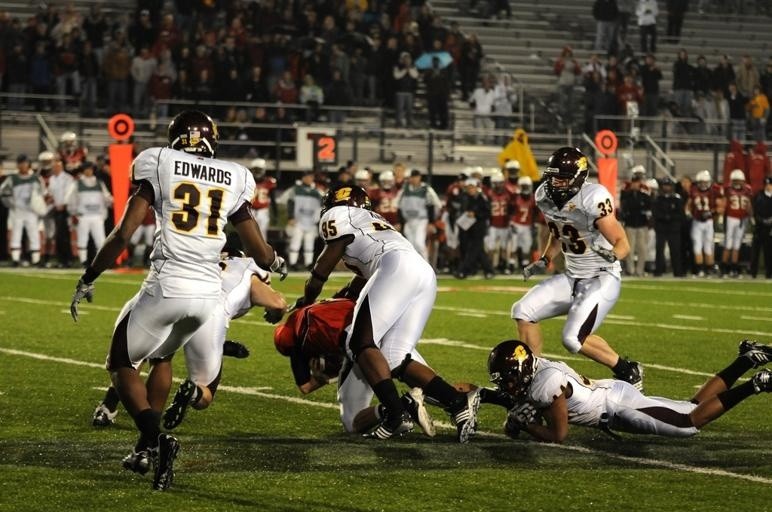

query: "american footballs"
left=307, top=351, right=342, bottom=379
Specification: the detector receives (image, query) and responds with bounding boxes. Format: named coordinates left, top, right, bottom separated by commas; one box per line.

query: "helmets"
left=764, top=177, right=772, bottom=185
left=464, top=179, right=477, bottom=186
left=80, top=161, right=96, bottom=171
left=378, top=170, right=395, bottom=181
left=490, top=172, right=505, bottom=183
left=356, top=170, right=368, bottom=179
left=319, top=184, right=371, bottom=217
left=461, top=167, right=472, bottom=178
left=37, top=152, right=55, bottom=170
left=518, top=177, right=531, bottom=186
left=633, top=164, right=646, bottom=173
left=15, top=155, right=31, bottom=165
left=471, top=167, right=483, bottom=177
left=696, top=170, right=711, bottom=183
left=507, top=160, right=520, bottom=169
left=59, top=131, right=77, bottom=145
left=646, top=179, right=658, bottom=190
left=249, top=159, right=265, bottom=169
left=338, top=167, right=352, bottom=176
left=404, top=168, right=420, bottom=178
left=730, top=169, right=745, bottom=181
left=543, top=147, right=588, bottom=200
left=632, top=172, right=645, bottom=181
left=488, top=341, right=535, bottom=403
left=661, top=176, right=672, bottom=185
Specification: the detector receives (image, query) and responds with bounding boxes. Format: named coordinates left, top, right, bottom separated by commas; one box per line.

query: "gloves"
left=270, top=251, right=288, bottom=282
left=522, top=256, right=549, bottom=281
left=223, top=341, right=249, bottom=358
left=71, top=277, right=95, bottom=321
left=591, top=243, right=617, bottom=263
left=701, top=211, right=713, bottom=222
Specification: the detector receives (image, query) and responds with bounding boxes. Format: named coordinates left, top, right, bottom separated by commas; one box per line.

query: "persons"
left=506, top=175, right=537, bottom=275
left=322, top=167, right=372, bottom=211
left=249, top=158, right=278, bottom=243
left=0, top=0, right=541, bottom=181
left=651, top=175, right=689, bottom=278
left=486, top=172, right=512, bottom=280
left=689, top=169, right=724, bottom=278
left=451, top=338, right=772, bottom=442
left=275, top=171, right=324, bottom=272
left=618, top=171, right=651, bottom=278
left=89, top=231, right=288, bottom=432
left=393, top=169, right=443, bottom=262
left=0, top=153, right=47, bottom=268
left=285, top=183, right=481, bottom=444
left=367, top=170, right=403, bottom=233
left=36, top=150, right=57, bottom=258
left=550, top=0, right=772, bottom=184
left=455, top=178, right=495, bottom=279
left=721, top=168, right=757, bottom=279
left=47, top=159, right=78, bottom=269
left=67, top=161, right=113, bottom=268
left=127, top=205, right=155, bottom=268
left=272, top=295, right=438, bottom=440
left=750, top=176, right=772, bottom=279
left=69, top=109, right=288, bottom=492
left=510, top=146, right=646, bottom=397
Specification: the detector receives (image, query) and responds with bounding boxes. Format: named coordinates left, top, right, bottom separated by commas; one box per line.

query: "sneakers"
left=733, top=272, right=743, bottom=279
left=400, top=387, right=436, bottom=437
left=612, top=360, right=643, bottom=394
left=504, top=266, right=513, bottom=275
left=752, top=369, right=772, bottom=395
left=738, top=341, right=772, bottom=368
left=508, top=403, right=543, bottom=426
left=449, top=389, right=481, bottom=443
left=93, top=400, right=119, bottom=428
left=162, top=378, right=197, bottom=429
left=455, top=273, right=468, bottom=278
left=289, top=263, right=301, bottom=273
left=693, top=271, right=703, bottom=279
left=705, top=273, right=712, bottom=279
left=362, top=412, right=415, bottom=440
left=146, top=433, right=179, bottom=492
left=720, top=274, right=728, bottom=278
left=485, top=273, right=494, bottom=279
left=121, top=446, right=153, bottom=475
left=304, top=265, right=312, bottom=272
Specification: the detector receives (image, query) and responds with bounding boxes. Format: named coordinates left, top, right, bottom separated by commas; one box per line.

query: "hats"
left=563, top=45, right=573, bottom=55
